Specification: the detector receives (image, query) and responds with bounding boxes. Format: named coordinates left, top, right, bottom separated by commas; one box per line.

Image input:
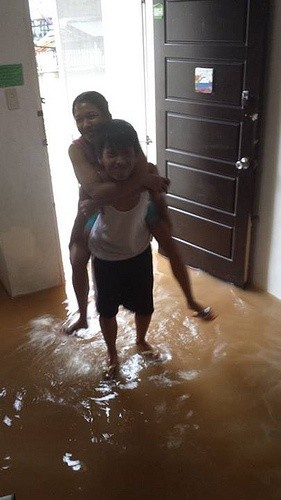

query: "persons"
left=61, top=92, right=219, bottom=333
left=71, top=121, right=172, bottom=380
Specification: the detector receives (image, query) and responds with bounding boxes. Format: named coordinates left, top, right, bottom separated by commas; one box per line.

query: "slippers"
left=190, top=307, right=217, bottom=322
left=55, top=325, right=77, bottom=341
